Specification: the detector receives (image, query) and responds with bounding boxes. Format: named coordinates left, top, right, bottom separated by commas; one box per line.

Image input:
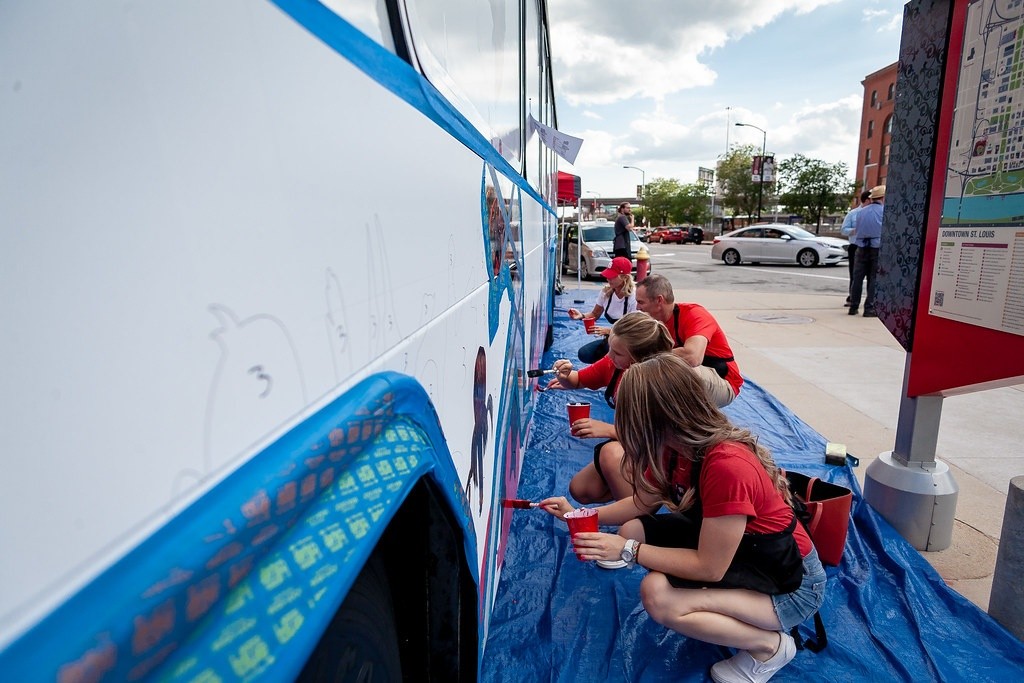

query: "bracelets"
left=632, top=541, right=642, bottom=565
left=580, top=314, right=585, bottom=320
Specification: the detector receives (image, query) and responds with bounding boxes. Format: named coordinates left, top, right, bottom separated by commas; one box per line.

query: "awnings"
left=558, top=170, right=581, bottom=207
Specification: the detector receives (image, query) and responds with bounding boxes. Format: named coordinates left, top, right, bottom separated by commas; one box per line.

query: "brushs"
left=554, top=307, right=567, bottom=312
left=500, top=499, right=558, bottom=509
left=527, top=369, right=567, bottom=377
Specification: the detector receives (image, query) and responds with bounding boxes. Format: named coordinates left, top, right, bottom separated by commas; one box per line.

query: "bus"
left=0, top=1, right=563, bottom=683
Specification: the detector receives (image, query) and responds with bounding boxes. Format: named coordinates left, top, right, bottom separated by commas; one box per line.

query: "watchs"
left=620, top=539, right=636, bottom=562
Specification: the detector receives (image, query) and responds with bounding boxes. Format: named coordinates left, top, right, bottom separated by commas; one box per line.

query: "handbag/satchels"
left=777, top=468, right=852, bottom=566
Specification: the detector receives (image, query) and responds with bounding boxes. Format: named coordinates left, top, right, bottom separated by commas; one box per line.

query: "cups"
left=566, top=401, right=592, bottom=436
left=562, top=508, right=599, bottom=561
left=582, top=316, right=595, bottom=334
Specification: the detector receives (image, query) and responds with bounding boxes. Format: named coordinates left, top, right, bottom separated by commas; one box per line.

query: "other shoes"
left=863, top=311, right=877, bottom=317
left=849, top=308, right=858, bottom=315
left=711, top=631, right=797, bottom=683
left=844, top=301, right=851, bottom=306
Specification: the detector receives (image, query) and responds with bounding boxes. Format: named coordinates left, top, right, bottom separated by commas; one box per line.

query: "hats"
left=600, top=257, right=632, bottom=279
left=868, top=185, right=886, bottom=198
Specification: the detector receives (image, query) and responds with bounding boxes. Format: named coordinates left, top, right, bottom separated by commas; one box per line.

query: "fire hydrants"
left=634, top=246, right=651, bottom=285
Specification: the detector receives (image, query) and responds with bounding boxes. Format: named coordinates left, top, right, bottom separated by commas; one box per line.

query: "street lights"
left=587, top=190, right=601, bottom=217
left=735, top=123, right=766, bottom=222
left=623, top=166, right=645, bottom=227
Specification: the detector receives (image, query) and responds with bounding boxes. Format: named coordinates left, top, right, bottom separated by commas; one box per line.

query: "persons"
left=547, top=274, right=744, bottom=408
left=540, top=355, right=826, bottom=683
left=569, top=257, right=638, bottom=363
left=842, top=185, right=886, bottom=317
left=552, top=311, right=675, bottom=569
left=613, top=202, right=634, bottom=261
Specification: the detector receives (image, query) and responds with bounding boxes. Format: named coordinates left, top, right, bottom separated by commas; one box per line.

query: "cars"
left=712, top=223, right=851, bottom=268
left=557, top=216, right=655, bottom=243
left=673, top=226, right=705, bottom=245
left=646, top=225, right=683, bottom=245
left=562, top=217, right=652, bottom=281
